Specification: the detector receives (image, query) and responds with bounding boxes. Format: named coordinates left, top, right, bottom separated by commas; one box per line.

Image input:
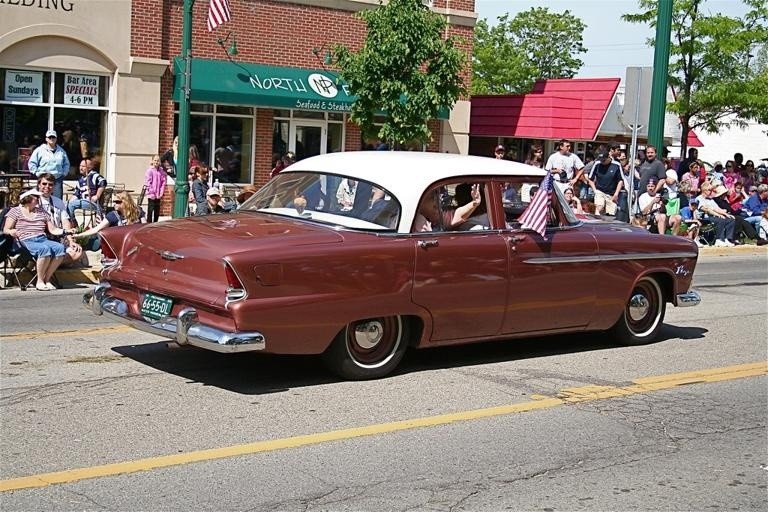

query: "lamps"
left=311, top=40, right=332, bottom=64
left=218, top=29, right=239, bottom=57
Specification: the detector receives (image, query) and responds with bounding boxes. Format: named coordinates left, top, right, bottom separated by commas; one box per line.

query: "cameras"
left=559, top=169, right=567, bottom=180
left=660, top=188, right=669, bottom=205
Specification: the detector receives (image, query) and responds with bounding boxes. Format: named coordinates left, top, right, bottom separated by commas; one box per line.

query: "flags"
left=207, top=0, right=231, bottom=34
left=517, top=171, right=554, bottom=241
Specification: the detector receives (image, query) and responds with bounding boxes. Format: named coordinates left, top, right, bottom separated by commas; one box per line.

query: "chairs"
left=0, top=172, right=151, bottom=293
left=215, top=186, right=255, bottom=207
left=627, top=193, right=766, bottom=246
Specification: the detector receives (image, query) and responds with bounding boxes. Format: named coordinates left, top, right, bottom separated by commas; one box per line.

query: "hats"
left=46, top=130, right=57, bottom=138
left=236, top=185, right=257, bottom=204
left=596, top=152, right=609, bottom=161
left=689, top=198, right=699, bottom=205
left=207, top=187, right=222, bottom=197
left=757, top=184, right=768, bottom=193
left=19, top=188, right=44, bottom=200
left=714, top=186, right=729, bottom=197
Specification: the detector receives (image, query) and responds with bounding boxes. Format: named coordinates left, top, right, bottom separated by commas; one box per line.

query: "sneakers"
left=693, top=236, right=767, bottom=247
left=37, top=282, right=56, bottom=291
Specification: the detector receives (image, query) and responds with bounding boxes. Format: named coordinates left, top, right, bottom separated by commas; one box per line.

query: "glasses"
left=39, top=181, right=54, bottom=186
left=112, top=200, right=122, bottom=205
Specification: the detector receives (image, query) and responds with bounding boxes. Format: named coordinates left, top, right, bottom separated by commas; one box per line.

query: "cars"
left=84, top=149, right=701, bottom=382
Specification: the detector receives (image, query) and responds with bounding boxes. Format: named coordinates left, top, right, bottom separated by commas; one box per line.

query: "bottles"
left=213, top=178, right=220, bottom=188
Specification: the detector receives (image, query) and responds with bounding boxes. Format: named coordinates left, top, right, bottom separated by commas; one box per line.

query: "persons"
left=525, top=139, right=768, bottom=247
left=269, top=153, right=321, bottom=210
left=413, top=182, right=514, bottom=233
left=188, top=145, right=257, bottom=216
left=495, top=144, right=505, bottom=160
left=2, top=128, right=179, bottom=291
left=336, top=178, right=358, bottom=213
